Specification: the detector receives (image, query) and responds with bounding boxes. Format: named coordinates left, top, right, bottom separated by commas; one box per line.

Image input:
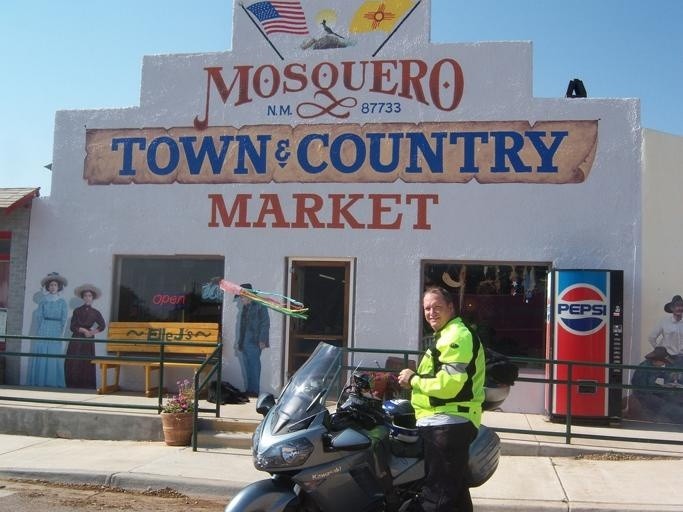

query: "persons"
left=63, top=282, right=105, bottom=390
left=396, top=285, right=486, bottom=511
left=631, top=346, right=682, bottom=424
left=24, top=271, right=67, bottom=387
left=646, top=294, right=682, bottom=385
left=232, top=282, right=271, bottom=397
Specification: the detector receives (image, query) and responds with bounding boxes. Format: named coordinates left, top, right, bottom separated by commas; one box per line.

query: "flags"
left=243, top=1, right=311, bottom=38
left=344, top=0, right=416, bottom=35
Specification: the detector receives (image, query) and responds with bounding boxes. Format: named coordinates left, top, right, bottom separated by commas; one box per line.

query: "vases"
left=160, top=414, right=195, bottom=446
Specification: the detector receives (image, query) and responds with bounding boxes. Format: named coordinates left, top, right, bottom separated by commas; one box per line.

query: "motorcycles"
left=224, top=339, right=520, bottom=512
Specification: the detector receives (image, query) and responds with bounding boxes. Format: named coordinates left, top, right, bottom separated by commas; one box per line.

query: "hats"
left=235, top=283, right=256, bottom=298
left=645, top=347, right=675, bottom=367
left=664, top=295, right=683, bottom=314
left=74, top=283, right=101, bottom=301
left=41, top=272, right=68, bottom=292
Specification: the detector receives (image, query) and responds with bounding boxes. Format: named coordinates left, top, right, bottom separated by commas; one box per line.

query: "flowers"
left=156, top=373, right=199, bottom=413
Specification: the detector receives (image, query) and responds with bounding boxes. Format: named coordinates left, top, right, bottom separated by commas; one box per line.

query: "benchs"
left=91, top=321, right=220, bottom=397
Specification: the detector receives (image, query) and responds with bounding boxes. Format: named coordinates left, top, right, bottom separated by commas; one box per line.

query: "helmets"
left=381, top=399, right=419, bottom=443
